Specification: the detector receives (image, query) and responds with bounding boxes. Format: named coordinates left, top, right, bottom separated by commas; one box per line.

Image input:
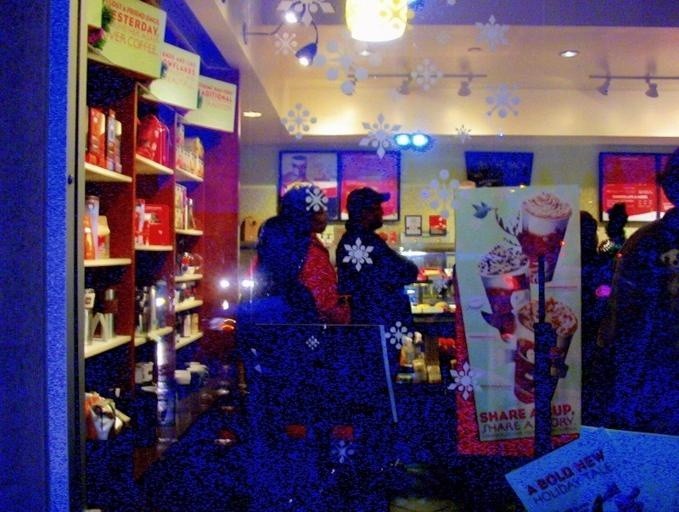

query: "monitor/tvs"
left=463, top=151, right=534, bottom=186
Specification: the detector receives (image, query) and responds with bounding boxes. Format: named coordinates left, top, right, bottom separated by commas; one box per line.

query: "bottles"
left=84, top=196, right=111, bottom=260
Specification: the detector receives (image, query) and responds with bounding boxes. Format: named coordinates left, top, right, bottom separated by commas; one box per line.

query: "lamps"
left=588, top=75, right=679, bottom=97
left=344, top=73, right=487, bottom=96
left=243, top=1, right=319, bottom=67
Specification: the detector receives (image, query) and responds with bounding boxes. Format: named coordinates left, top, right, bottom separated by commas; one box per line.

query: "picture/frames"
left=658, top=152, right=674, bottom=222
left=598, top=151, right=658, bottom=222
left=429, top=215, right=447, bottom=235
left=277, top=149, right=339, bottom=222
left=405, top=215, right=422, bottom=236
left=339, top=149, right=400, bottom=222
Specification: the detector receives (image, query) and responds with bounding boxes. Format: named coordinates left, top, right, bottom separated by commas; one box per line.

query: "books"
left=503, top=426, right=663, bottom=511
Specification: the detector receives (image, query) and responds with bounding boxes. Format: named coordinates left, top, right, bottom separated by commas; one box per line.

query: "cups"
left=480, top=202, right=578, bottom=403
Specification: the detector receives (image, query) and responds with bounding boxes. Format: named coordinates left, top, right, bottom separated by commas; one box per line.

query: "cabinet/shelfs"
left=0, top=0, right=239, bottom=512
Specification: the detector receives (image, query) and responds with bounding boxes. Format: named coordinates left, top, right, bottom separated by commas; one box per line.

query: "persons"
left=277, top=154, right=311, bottom=196
left=579, top=146, right=679, bottom=436
left=232, top=210, right=327, bottom=508
left=333, top=184, right=420, bottom=370
left=275, top=186, right=357, bottom=329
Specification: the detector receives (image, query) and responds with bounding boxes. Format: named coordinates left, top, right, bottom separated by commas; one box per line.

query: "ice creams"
left=477, top=243, right=526, bottom=275
left=513, top=296, right=576, bottom=335
left=522, top=190, right=571, bottom=219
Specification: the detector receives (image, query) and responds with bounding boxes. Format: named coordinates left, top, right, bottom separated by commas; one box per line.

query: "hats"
left=345, top=186, right=392, bottom=216
